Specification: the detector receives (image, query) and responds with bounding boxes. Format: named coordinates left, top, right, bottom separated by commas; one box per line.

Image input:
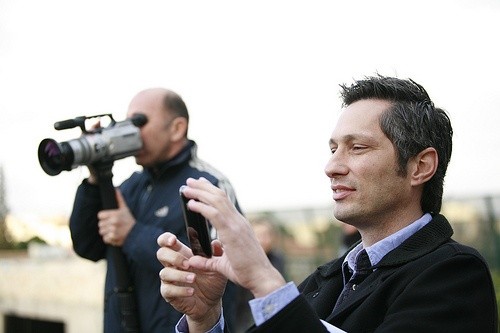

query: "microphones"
left=54, top=117, right=82, bottom=130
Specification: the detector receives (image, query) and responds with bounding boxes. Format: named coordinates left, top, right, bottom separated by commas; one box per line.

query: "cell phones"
left=179, top=185, right=213, bottom=258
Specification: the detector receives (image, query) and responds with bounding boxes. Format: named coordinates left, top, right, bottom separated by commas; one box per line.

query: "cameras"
left=38, top=114, right=145, bottom=176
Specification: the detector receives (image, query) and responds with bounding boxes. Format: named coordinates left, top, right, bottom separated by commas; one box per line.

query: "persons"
left=155, top=73, right=500, bottom=333
left=68, top=88, right=247, bottom=333
